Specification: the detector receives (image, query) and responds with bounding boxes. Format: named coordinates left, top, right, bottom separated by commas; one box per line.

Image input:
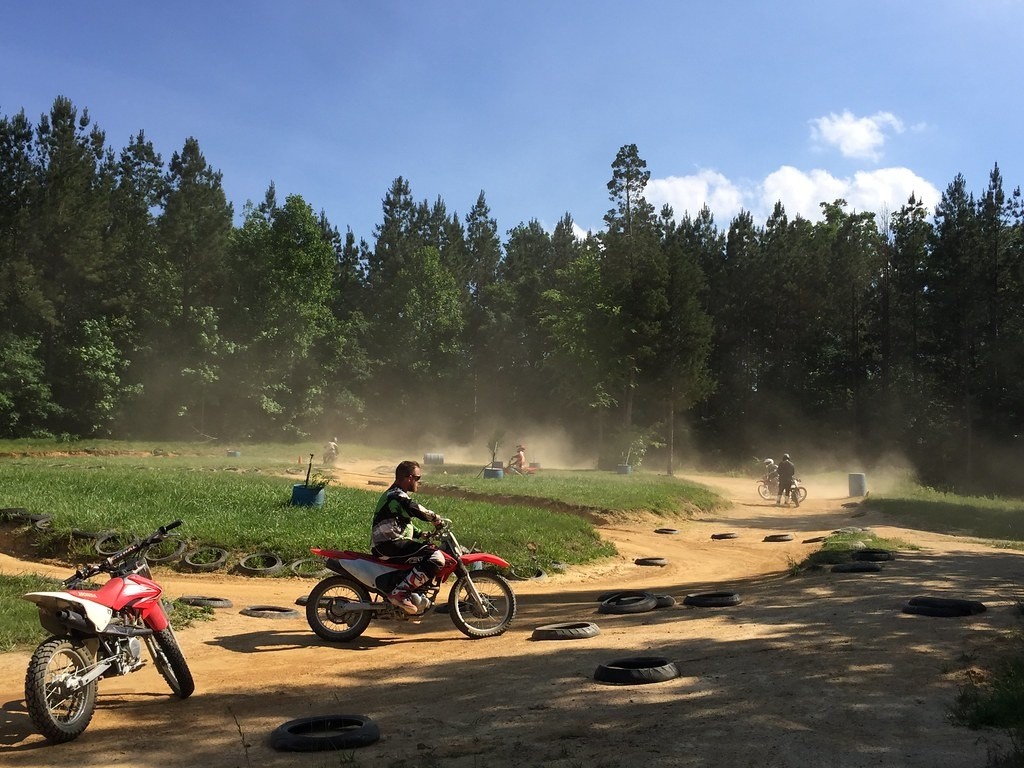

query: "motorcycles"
left=790, top=474, right=807, bottom=508
left=324, top=446, right=340, bottom=465
left=504, top=455, right=539, bottom=477
left=304, top=513, right=517, bottom=637
left=756, top=472, right=799, bottom=500
left=19, top=518, right=197, bottom=743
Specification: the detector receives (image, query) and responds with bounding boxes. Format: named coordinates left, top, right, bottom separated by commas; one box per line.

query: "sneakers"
left=387, top=588, right=418, bottom=614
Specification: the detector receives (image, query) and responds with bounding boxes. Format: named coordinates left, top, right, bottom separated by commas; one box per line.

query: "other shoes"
left=775, top=501, right=780, bottom=507
left=782, top=502, right=791, bottom=507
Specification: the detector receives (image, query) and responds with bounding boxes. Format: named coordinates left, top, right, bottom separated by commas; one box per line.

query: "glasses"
left=406, top=475, right=421, bottom=481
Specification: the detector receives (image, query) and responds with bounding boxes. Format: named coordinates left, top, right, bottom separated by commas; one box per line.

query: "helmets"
left=783, top=454, right=789, bottom=461
left=517, top=445, right=525, bottom=452
left=331, top=437, right=337, bottom=443
left=764, top=459, right=774, bottom=468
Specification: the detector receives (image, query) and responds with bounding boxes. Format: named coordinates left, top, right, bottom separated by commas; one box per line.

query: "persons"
left=764, top=457, right=780, bottom=488
left=371, top=461, right=442, bottom=625
left=512, top=444, right=525, bottom=472
left=776, top=453, right=795, bottom=507
left=323, top=436, right=339, bottom=461
